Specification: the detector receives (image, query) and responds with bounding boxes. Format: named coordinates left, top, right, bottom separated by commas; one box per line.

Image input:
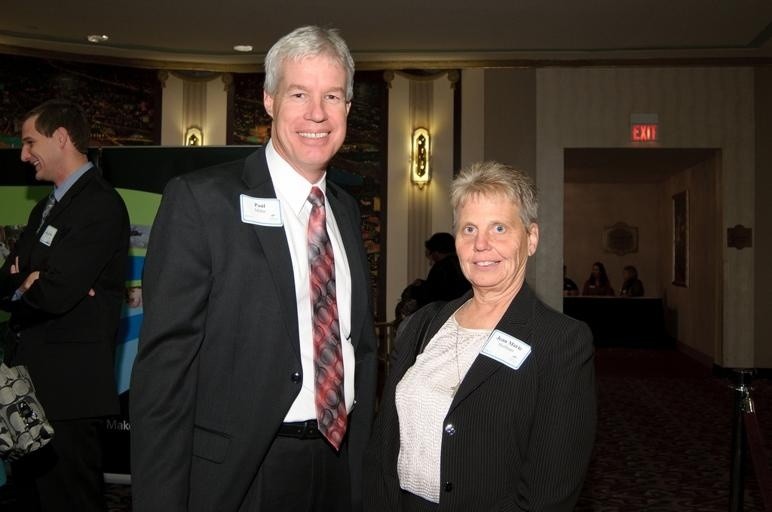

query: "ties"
left=309, top=186, right=348, bottom=453
left=37, top=195, right=57, bottom=232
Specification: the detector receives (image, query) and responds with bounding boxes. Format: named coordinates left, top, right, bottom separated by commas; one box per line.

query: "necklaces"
left=449, top=300, right=472, bottom=399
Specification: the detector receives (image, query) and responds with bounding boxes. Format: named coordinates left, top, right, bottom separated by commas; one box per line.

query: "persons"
left=415, top=232, right=472, bottom=311
left=564, top=266, right=578, bottom=296
left=362, top=161, right=597, bottom=512
left=0, top=98, right=131, bottom=512
left=129, top=26, right=378, bottom=512
left=619, top=266, right=644, bottom=297
left=582, top=262, right=615, bottom=296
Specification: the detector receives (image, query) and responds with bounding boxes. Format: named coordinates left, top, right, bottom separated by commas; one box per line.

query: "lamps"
left=184, top=125, right=202, bottom=146
left=410, top=127, right=432, bottom=189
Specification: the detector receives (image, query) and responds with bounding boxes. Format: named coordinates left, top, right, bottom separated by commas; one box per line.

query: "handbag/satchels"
left=0, top=361, right=57, bottom=463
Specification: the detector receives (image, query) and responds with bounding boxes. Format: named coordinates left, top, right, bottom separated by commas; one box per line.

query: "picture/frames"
left=672, top=189, right=690, bottom=288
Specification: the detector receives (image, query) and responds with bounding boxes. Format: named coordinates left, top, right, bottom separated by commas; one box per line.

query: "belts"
left=276, top=419, right=325, bottom=441
